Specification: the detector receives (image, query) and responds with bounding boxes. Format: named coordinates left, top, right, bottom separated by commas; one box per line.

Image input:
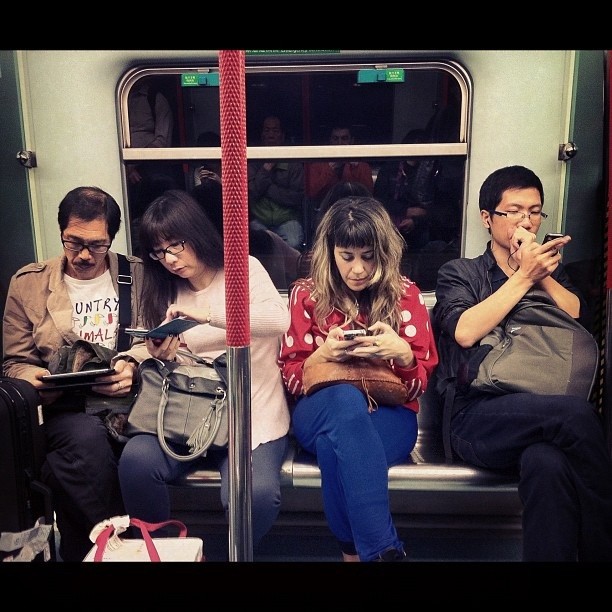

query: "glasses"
left=60, top=235, right=113, bottom=254
left=492, top=211, right=548, bottom=226
left=149, top=239, right=187, bottom=260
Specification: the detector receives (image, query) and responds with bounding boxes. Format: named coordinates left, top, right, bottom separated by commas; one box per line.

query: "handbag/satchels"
left=284, top=279, right=408, bottom=413
left=129, top=349, right=231, bottom=459
left=84, top=517, right=203, bottom=561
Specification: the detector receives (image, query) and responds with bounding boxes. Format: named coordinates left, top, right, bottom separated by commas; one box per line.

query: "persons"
left=130, top=80, right=174, bottom=217
left=435, top=164, right=610, bottom=562
left=247, top=114, right=304, bottom=290
left=275, top=193, right=440, bottom=564
left=0, top=185, right=154, bottom=563
left=119, top=192, right=291, bottom=563
left=377, top=130, right=452, bottom=244
left=302, top=125, right=376, bottom=234
left=192, top=132, right=228, bottom=237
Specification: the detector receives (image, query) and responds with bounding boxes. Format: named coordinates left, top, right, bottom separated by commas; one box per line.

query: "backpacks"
left=466, top=291, right=598, bottom=400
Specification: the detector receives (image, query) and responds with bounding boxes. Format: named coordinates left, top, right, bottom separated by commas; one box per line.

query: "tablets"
left=42, top=368, right=116, bottom=382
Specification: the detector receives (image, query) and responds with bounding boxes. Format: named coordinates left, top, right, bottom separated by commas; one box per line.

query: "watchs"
left=206, top=307, right=211, bottom=323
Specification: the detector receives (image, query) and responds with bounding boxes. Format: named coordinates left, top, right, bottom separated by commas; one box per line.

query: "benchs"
left=168, top=293, right=523, bottom=515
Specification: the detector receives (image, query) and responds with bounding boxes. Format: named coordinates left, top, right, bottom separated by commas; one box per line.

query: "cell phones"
left=542, top=232, right=564, bottom=257
left=343, top=329, right=367, bottom=341
left=123, top=328, right=150, bottom=336
